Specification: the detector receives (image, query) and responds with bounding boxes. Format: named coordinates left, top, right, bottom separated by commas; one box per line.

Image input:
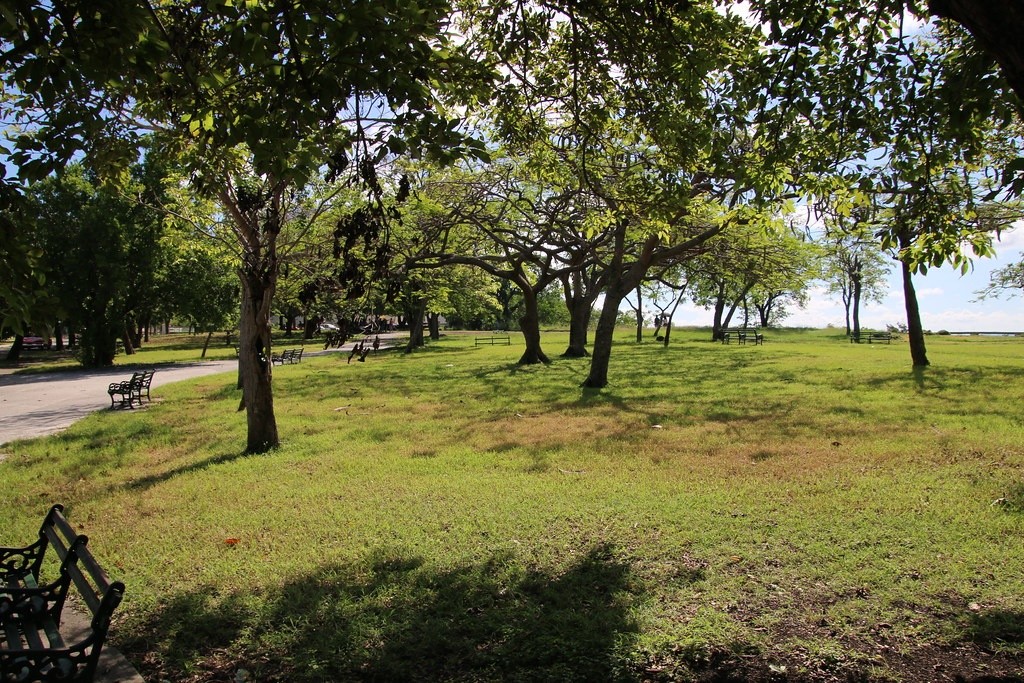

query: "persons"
left=655, top=315, right=660, bottom=326
left=662, top=316, right=667, bottom=326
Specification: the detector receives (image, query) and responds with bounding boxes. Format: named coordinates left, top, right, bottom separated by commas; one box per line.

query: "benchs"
left=0, top=503, right=126, bottom=683
left=720, top=328, right=763, bottom=345
left=272, top=347, right=305, bottom=365
left=850, top=330, right=892, bottom=345
left=234, top=345, right=239, bottom=359
left=108, top=368, right=156, bottom=408
left=21, top=337, right=52, bottom=350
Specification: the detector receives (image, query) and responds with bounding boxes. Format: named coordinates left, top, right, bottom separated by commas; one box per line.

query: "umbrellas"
left=659, top=313, right=670, bottom=317
